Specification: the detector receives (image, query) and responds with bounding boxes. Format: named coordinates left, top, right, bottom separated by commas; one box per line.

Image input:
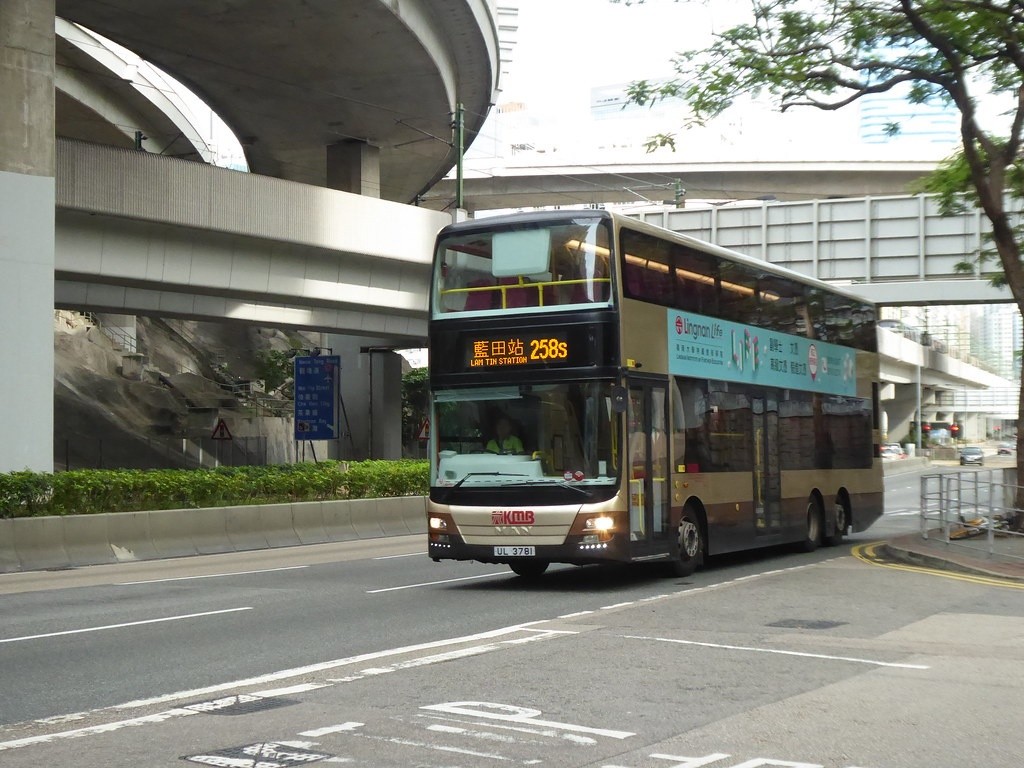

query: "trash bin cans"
left=905, top=443, right=916, bottom=459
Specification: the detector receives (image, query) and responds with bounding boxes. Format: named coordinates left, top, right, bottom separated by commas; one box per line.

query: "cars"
left=881, top=443, right=903, bottom=460
left=908, top=419, right=960, bottom=443
left=958, top=446, right=984, bottom=467
left=997, top=444, right=1011, bottom=455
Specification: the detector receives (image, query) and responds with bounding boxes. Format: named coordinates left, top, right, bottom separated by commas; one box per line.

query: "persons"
left=482, top=414, right=524, bottom=455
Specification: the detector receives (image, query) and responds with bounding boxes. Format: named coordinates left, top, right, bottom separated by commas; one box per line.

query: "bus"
left=425, top=208, right=885, bottom=580
